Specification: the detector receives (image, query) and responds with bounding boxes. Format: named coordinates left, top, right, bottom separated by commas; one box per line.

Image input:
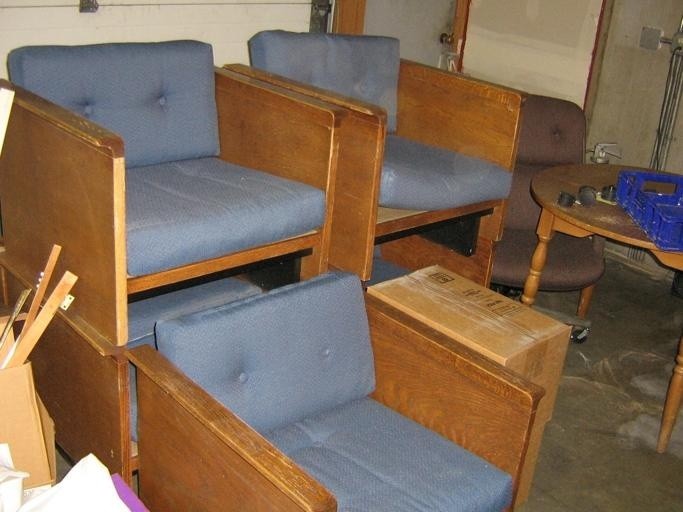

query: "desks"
left=521, top=163, right=683, bottom=454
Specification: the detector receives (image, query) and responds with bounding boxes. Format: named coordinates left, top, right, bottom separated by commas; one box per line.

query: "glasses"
left=557, top=189, right=596, bottom=209
left=578, top=184, right=617, bottom=201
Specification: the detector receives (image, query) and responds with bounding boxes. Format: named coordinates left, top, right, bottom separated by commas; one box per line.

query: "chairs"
left=482, top=89, right=603, bottom=313
left=2, top=30, right=338, bottom=490
left=126, top=272, right=542, bottom=510
left=223, top=32, right=527, bottom=295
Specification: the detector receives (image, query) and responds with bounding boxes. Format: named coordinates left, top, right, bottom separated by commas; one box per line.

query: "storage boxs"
left=365, top=266, right=573, bottom=427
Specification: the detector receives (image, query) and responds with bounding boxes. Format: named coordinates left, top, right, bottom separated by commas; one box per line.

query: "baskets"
left=618, top=169, right=683, bottom=251
